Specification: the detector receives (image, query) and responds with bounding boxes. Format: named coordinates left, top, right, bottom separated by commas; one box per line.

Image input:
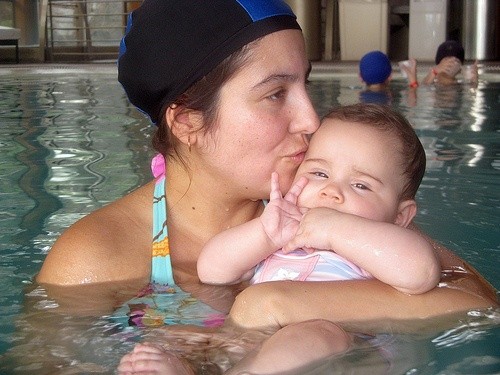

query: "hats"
left=360, top=51, right=392, bottom=83
left=435, top=40, right=465, bottom=65
left=117, top=0, right=302, bottom=127
left=360, top=89, right=388, bottom=105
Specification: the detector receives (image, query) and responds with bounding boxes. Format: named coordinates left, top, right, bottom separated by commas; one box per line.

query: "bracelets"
left=432, top=68, right=438, bottom=75
left=408, top=81, right=418, bottom=88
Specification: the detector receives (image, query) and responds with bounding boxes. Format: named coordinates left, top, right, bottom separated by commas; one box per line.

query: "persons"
left=421, top=41, right=480, bottom=89
left=355, top=52, right=421, bottom=99
left=114, top=103, right=444, bottom=375
left=30, top=1, right=500, bottom=321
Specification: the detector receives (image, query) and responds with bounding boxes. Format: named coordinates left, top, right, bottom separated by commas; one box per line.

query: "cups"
left=460, top=64, right=476, bottom=83
left=398, top=60, right=412, bottom=79
left=444, top=63, right=459, bottom=77
